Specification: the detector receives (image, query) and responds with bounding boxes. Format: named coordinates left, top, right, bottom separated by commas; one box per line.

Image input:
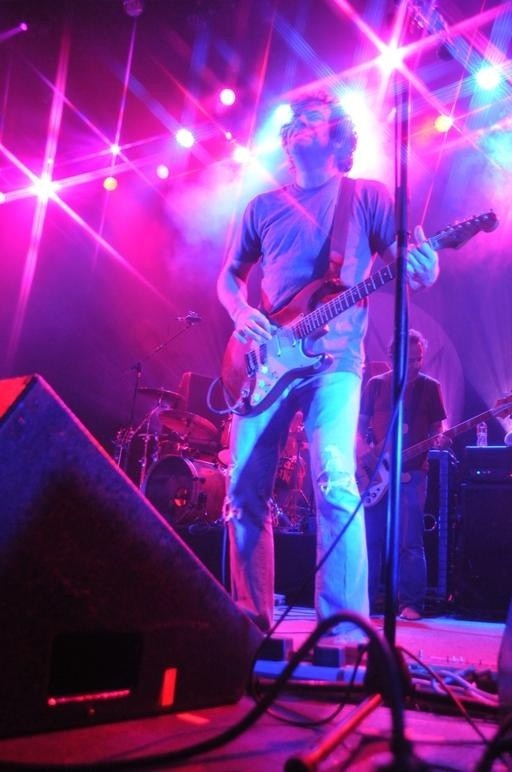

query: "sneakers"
left=399, top=606, right=420, bottom=621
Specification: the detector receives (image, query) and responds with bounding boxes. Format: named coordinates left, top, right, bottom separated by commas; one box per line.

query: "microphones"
left=175, top=316, right=202, bottom=323
left=407, top=3, right=457, bottom=62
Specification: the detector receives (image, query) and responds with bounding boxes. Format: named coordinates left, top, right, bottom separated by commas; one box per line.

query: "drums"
left=218, top=410, right=234, bottom=464
left=143, top=455, right=230, bottom=529
left=276, top=457, right=306, bottom=493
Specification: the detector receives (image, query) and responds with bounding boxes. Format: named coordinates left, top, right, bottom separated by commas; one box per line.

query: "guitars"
left=356, top=390, right=512, bottom=508
left=220, top=208, right=500, bottom=417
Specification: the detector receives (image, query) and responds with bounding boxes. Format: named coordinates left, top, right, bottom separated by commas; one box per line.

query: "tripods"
left=251, top=77, right=511, bottom=771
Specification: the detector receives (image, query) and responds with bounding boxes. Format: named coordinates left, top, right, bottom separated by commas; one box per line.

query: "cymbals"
left=157, top=407, right=218, bottom=444
left=134, top=385, right=185, bottom=406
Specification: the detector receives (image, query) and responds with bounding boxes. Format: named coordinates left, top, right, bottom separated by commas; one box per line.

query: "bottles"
left=472, top=422, right=488, bottom=447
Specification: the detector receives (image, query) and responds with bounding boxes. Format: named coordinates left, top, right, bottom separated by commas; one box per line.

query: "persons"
left=356, top=327, right=454, bottom=624
left=214, top=90, right=441, bottom=661
